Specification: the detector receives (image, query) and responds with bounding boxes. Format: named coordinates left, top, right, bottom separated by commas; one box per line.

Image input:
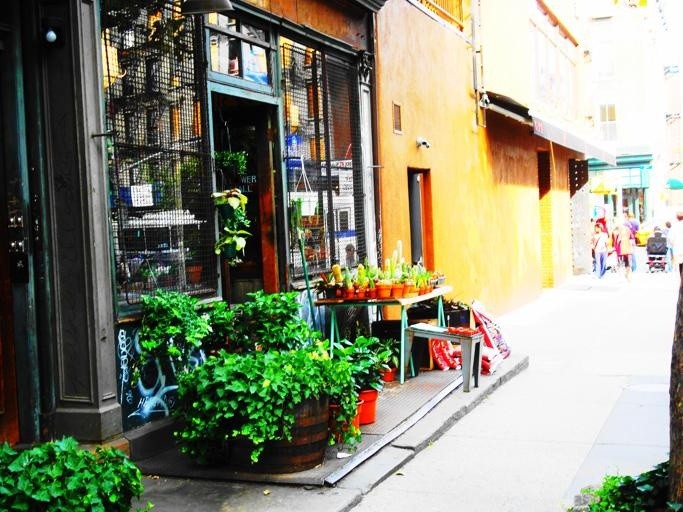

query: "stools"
left=402, top=322, right=484, bottom=392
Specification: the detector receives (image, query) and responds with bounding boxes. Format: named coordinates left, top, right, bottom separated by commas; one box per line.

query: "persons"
left=586, top=201, right=682, bottom=287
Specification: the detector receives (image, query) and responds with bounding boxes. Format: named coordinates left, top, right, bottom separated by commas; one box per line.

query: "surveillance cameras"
left=415, top=136, right=430, bottom=149
